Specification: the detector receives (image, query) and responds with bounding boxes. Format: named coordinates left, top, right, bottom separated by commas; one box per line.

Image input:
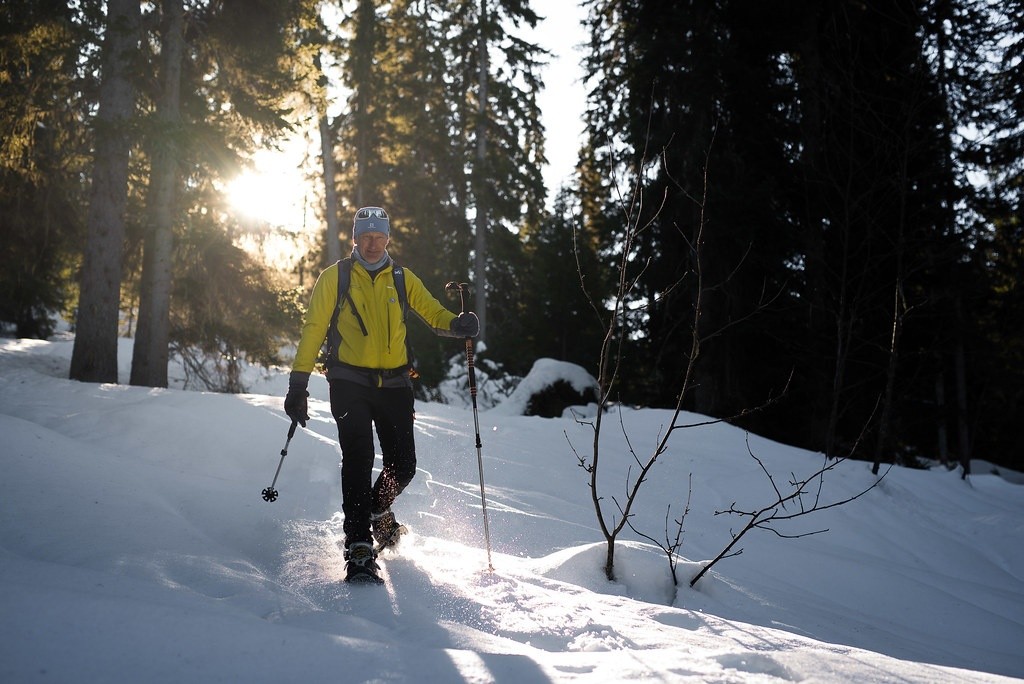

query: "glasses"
left=354, top=208, right=389, bottom=226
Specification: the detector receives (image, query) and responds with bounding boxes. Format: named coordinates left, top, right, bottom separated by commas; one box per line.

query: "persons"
left=284, top=206, right=480, bottom=584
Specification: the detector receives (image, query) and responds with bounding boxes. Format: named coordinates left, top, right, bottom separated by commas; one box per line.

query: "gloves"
left=285, top=371, right=310, bottom=428
left=450, top=312, right=479, bottom=338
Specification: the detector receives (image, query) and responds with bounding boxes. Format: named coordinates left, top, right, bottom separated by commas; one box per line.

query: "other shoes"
left=370, top=487, right=400, bottom=548
left=346, top=542, right=374, bottom=582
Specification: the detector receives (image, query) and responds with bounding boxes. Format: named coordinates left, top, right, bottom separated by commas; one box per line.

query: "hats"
left=353, top=207, right=389, bottom=238
